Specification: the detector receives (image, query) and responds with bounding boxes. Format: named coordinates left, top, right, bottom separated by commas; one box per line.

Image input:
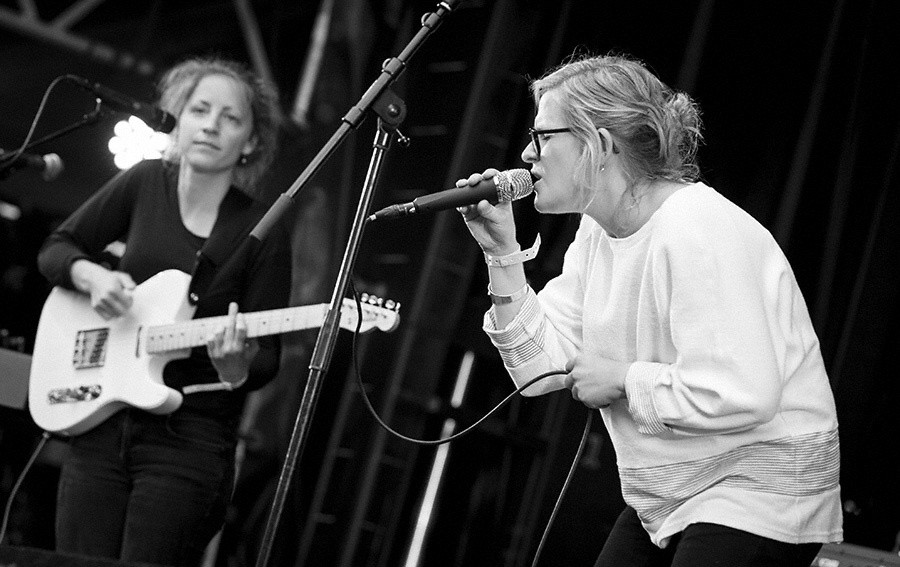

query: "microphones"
left=368, top=167, right=534, bottom=223
left=0, top=150, right=67, bottom=184
left=67, top=73, right=178, bottom=135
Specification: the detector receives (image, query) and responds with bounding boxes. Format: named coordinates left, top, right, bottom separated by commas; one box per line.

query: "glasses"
left=529, top=127, right=570, bottom=159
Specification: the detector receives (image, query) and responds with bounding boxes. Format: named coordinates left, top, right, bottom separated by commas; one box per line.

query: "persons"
left=455, top=57, right=844, bottom=567
left=37, top=57, right=294, bottom=567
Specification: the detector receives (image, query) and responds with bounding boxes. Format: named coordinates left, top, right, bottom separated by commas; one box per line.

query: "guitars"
left=27, top=266, right=404, bottom=437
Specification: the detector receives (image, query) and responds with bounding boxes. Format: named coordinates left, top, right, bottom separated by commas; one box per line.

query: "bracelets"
left=485, top=234, right=542, bottom=269
left=487, top=282, right=529, bottom=306
